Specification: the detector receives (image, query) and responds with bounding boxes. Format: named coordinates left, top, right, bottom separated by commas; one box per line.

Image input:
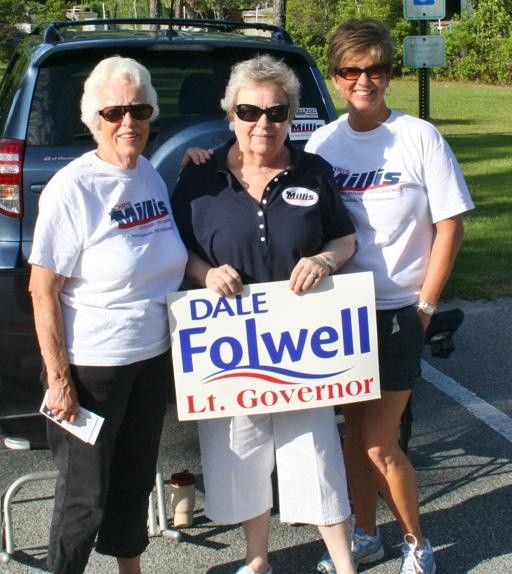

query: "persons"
left=27, top=53, right=214, bottom=573
left=301, top=15, right=475, bottom=572
left=172, top=53, right=361, bottom=573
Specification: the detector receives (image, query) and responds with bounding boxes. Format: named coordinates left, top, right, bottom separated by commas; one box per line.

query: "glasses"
left=333, top=63, right=387, bottom=80
left=95, top=103, right=153, bottom=123
left=232, top=103, right=291, bottom=124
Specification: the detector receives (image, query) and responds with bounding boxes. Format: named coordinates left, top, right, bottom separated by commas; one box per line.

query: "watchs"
left=415, top=298, right=435, bottom=316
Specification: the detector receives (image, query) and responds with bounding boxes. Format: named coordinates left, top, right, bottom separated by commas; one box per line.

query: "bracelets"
left=320, top=254, right=337, bottom=273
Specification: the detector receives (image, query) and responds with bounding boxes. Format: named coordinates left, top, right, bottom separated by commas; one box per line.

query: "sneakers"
left=318, top=526, right=387, bottom=573
left=399, top=538, right=437, bottom=574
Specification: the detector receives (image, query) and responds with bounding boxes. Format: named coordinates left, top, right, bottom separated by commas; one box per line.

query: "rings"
left=310, top=271, right=318, bottom=280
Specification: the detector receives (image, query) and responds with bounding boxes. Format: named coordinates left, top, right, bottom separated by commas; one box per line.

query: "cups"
left=170, top=468, right=196, bottom=529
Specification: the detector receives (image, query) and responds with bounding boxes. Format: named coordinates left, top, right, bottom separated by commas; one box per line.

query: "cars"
left=0, top=19, right=340, bottom=385
